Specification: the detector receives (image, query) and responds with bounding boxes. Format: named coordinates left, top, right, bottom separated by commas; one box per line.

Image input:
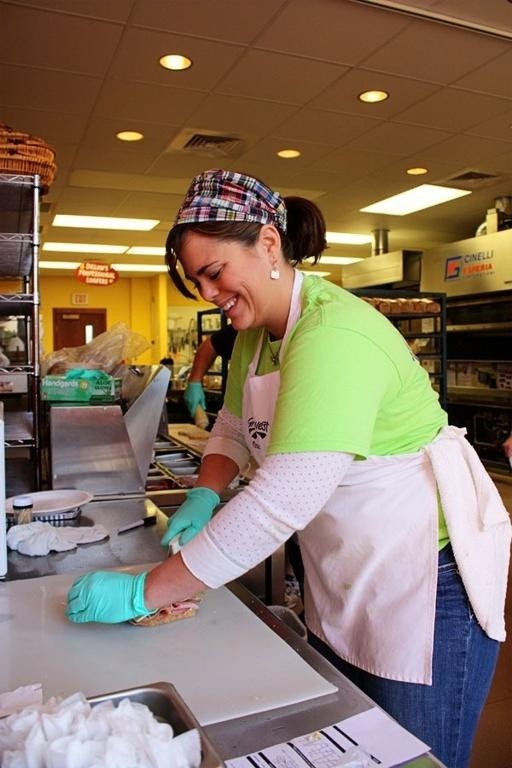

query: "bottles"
left=195, top=404, right=210, bottom=429
left=12, top=495, right=32, bottom=525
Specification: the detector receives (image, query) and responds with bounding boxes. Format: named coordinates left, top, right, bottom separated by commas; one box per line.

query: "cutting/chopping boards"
left=0, top=557, right=339, bottom=730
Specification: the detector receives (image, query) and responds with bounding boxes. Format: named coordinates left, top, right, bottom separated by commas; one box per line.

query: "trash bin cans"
left=267, top=605, right=308, bottom=643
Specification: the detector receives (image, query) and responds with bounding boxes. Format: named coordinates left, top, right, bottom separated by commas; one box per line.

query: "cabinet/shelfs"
left=196, top=288, right=448, bottom=426
left=0, top=169, right=41, bottom=494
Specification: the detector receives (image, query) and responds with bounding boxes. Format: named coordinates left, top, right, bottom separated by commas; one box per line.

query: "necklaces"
left=265, top=336, right=282, bottom=365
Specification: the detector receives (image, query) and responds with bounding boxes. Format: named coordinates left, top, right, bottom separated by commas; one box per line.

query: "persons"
left=65, top=165, right=512, bottom=768
left=183, top=316, right=302, bottom=612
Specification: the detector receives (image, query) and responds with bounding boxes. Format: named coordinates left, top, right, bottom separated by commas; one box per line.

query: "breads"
left=363, top=297, right=441, bottom=315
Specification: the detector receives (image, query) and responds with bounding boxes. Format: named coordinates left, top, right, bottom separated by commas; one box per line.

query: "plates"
left=5, top=488, right=93, bottom=515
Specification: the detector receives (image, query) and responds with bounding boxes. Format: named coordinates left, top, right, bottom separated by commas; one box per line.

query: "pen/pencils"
left=117, top=516, right=157, bottom=533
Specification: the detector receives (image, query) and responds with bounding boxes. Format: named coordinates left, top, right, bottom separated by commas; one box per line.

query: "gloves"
left=160, top=486, right=220, bottom=552
left=64, top=569, right=156, bottom=625
left=184, top=383, right=207, bottom=418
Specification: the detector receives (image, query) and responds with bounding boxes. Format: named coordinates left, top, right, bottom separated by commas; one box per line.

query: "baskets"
left=0, top=128, right=58, bottom=185
